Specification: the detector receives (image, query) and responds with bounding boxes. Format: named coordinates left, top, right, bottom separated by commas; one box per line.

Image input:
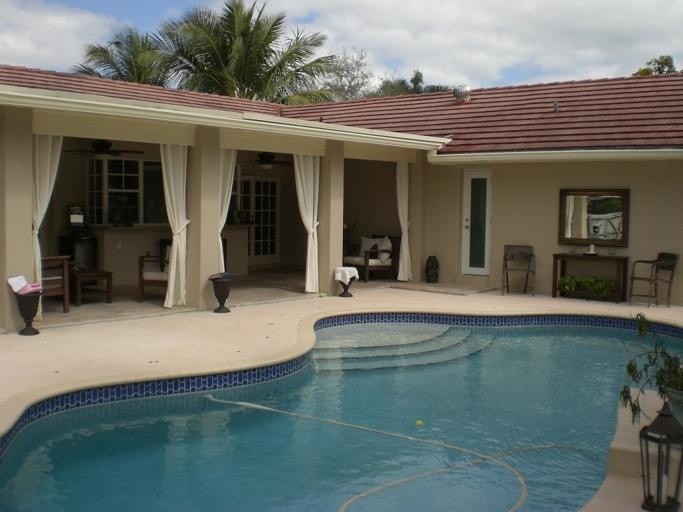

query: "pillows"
left=376, top=235, right=394, bottom=263
left=358, top=237, right=382, bottom=258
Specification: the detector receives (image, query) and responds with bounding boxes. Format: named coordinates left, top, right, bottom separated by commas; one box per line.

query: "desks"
left=551, top=253, right=629, bottom=303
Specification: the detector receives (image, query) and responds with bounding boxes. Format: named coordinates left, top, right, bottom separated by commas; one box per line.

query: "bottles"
left=591, top=226, right=599, bottom=254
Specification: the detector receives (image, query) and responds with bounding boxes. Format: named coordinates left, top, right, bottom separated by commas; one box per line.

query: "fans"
left=234, top=151, right=296, bottom=170
left=61, top=137, right=145, bottom=159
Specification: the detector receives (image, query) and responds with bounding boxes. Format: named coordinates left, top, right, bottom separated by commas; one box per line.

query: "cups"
left=607, top=244, right=616, bottom=255
left=570, top=242, right=576, bottom=254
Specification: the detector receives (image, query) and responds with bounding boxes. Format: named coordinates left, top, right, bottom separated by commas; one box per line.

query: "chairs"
left=501, top=243, right=537, bottom=296
left=342, top=233, right=401, bottom=283
left=68, top=236, right=115, bottom=306
left=134, top=237, right=172, bottom=305
left=627, top=251, right=680, bottom=309
left=40, top=253, right=72, bottom=314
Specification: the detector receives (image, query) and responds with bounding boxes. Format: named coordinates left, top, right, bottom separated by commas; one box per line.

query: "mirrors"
left=556, top=187, right=630, bottom=248
